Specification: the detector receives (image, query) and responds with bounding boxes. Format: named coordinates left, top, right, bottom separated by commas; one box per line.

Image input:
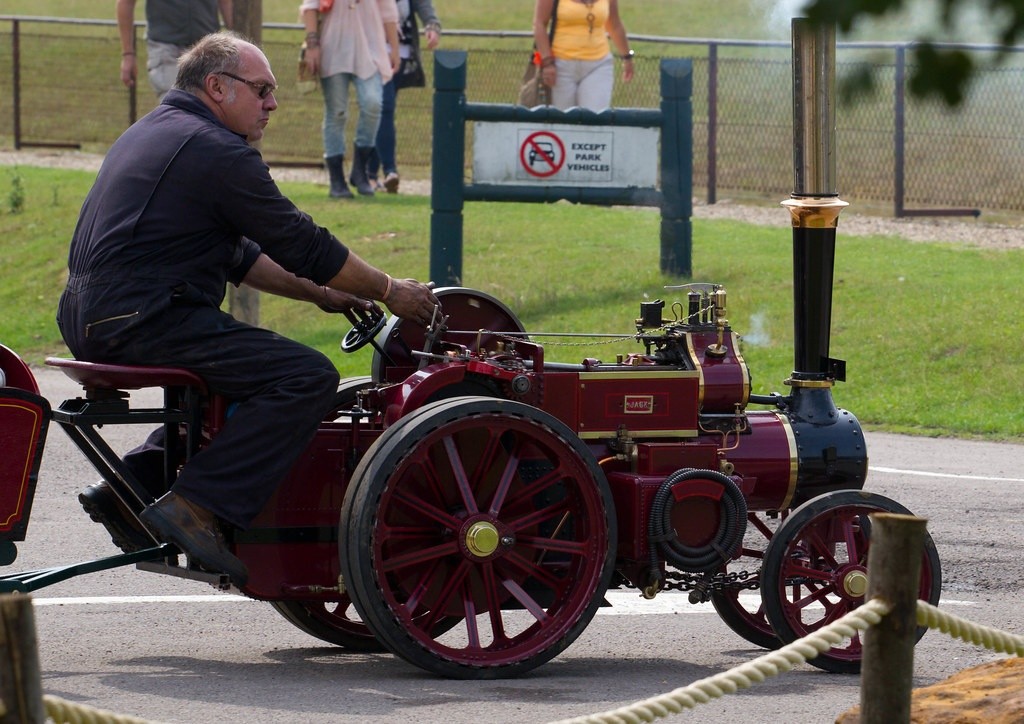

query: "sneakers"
left=140, top=492, right=254, bottom=583
left=78, top=471, right=164, bottom=563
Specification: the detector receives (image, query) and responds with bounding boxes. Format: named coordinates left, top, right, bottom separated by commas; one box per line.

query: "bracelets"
left=121, top=52, right=137, bottom=58
left=380, top=273, right=392, bottom=302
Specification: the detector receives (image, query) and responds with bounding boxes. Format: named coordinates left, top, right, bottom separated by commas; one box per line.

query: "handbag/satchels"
left=300, top=38, right=320, bottom=82
left=517, top=55, right=552, bottom=108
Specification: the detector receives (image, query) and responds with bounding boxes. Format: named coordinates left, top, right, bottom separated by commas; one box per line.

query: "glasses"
left=214, top=70, right=274, bottom=100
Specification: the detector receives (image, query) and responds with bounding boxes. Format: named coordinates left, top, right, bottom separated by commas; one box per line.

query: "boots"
left=325, top=154, right=354, bottom=198
left=349, top=143, right=373, bottom=196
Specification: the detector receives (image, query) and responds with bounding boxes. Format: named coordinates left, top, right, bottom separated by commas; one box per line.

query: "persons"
left=118, top=0, right=235, bottom=104
left=56, top=30, right=444, bottom=588
left=534, top=0, right=636, bottom=114
left=300, top=0, right=442, bottom=198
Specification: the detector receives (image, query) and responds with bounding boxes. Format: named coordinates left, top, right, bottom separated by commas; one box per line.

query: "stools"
left=44, top=355, right=206, bottom=399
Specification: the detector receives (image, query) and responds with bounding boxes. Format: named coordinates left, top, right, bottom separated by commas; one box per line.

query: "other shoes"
left=385, top=172, right=398, bottom=194
left=368, top=173, right=380, bottom=192
